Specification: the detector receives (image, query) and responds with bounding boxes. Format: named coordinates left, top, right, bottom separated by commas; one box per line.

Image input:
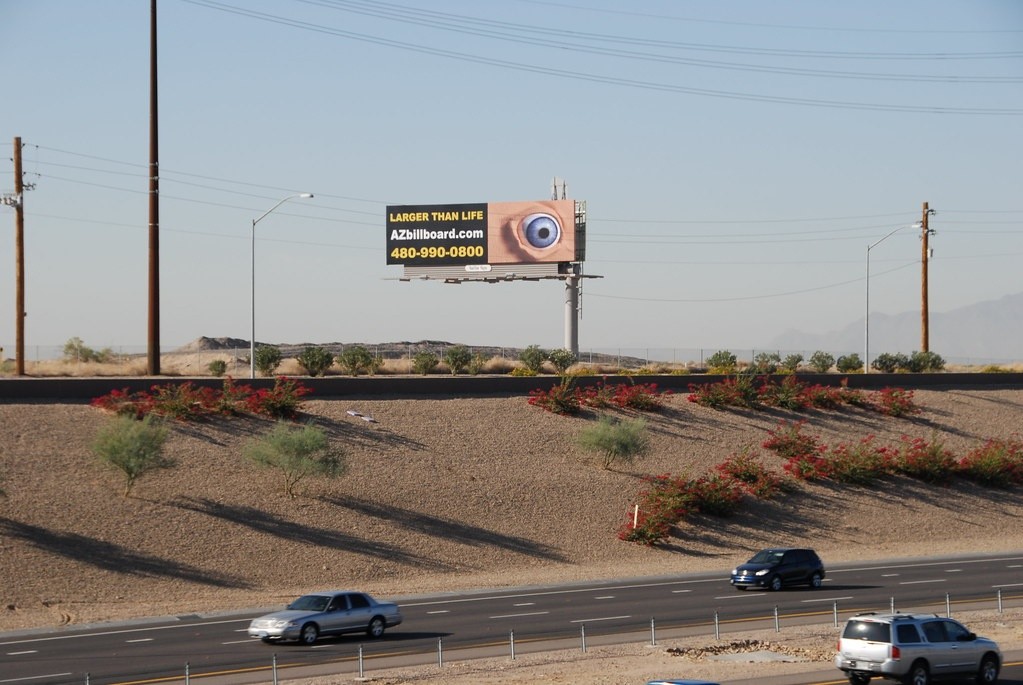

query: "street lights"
left=865, top=223, right=923, bottom=374
left=251, top=193, right=315, bottom=376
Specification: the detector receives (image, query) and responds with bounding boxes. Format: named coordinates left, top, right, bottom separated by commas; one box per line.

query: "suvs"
left=835, top=612, right=1003, bottom=685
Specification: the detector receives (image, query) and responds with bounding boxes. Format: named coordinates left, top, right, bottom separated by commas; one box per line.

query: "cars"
left=730, top=547, right=825, bottom=592
left=247, top=590, right=403, bottom=647
